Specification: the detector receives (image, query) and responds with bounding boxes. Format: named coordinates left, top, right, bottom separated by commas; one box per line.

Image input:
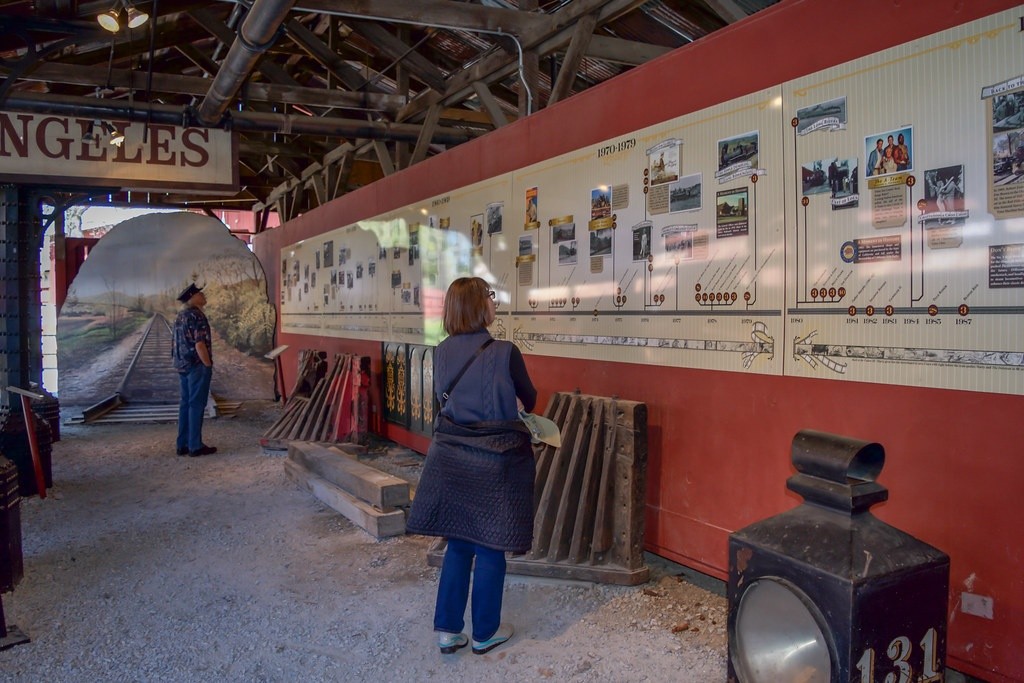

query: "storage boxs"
left=0, top=400, right=63, bottom=496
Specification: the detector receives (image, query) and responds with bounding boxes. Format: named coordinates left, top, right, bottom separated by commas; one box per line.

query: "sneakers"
left=437, top=631, right=469, bottom=653
left=472, top=624, right=513, bottom=655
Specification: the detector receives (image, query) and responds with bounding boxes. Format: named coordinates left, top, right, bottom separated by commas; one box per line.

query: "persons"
left=867, top=133, right=956, bottom=224
left=171, top=284, right=218, bottom=457
left=639, top=228, right=647, bottom=259
left=936, top=92, right=1024, bottom=225
left=404, top=277, right=538, bottom=654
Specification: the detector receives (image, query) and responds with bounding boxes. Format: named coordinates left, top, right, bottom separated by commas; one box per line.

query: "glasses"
left=487, top=291, right=495, bottom=299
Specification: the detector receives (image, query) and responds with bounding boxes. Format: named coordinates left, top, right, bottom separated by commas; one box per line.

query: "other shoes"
left=189, top=444, right=217, bottom=457
left=177, top=447, right=189, bottom=456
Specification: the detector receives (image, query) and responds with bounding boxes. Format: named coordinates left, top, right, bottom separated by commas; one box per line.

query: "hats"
left=176, top=282, right=203, bottom=303
left=517, top=410, right=562, bottom=448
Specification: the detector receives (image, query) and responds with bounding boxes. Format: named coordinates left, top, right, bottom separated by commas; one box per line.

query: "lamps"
left=97, top=0, right=123, bottom=32
left=104, top=121, right=125, bottom=147
left=122, top=0, right=149, bottom=29
left=81, top=121, right=96, bottom=145
